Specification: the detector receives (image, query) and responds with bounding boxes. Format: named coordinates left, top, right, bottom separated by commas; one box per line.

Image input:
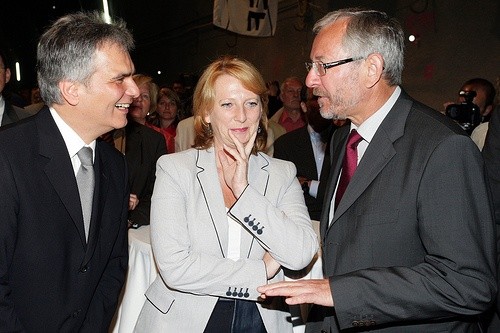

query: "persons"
left=0, top=12, right=140, bottom=333
left=131, top=55, right=318, bottom=333
left=98, top=74, right=350, bottom=230
left=262, top=10, right=496, bottom=333
left=0, top=56, right=46, bottom=126
left=440, top=77, right=500, bottom=224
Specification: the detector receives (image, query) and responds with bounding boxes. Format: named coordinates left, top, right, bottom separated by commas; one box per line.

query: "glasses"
left=305, top=56, right=385, bottom=77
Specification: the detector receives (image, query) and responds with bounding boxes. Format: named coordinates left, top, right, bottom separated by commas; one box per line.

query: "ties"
left=334, top=129, right=364, bottom=215
left=76, top=146, right=94, bottom=244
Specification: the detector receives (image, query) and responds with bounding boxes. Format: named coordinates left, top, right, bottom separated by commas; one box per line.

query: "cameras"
left=446, top=90, right=480, bottom=135
